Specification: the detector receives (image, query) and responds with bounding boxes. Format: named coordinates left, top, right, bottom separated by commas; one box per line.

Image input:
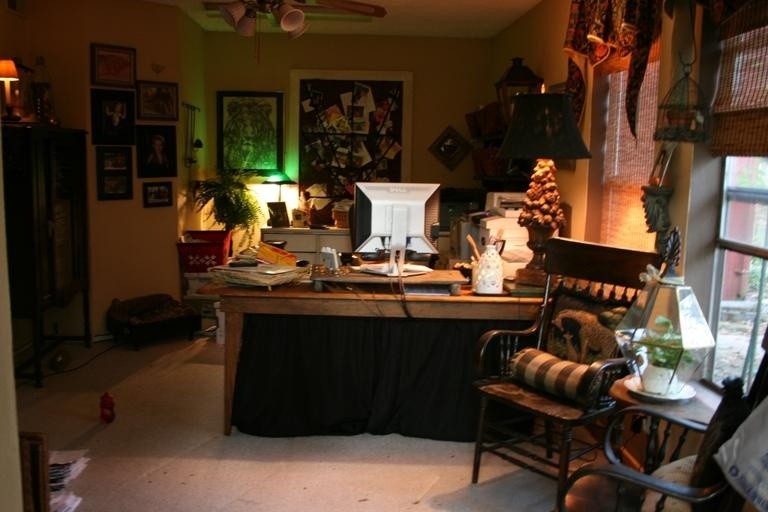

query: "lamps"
left=203, top=1, right=388, bottom=62
left=263, top=173, right=296, bottom=203
left=0, top=58, right=21, bottom=122
left=496, top=91, right=593, bottom=289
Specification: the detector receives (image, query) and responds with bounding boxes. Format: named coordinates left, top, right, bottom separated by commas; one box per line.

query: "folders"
left=207, top=264, right=299, bottom=287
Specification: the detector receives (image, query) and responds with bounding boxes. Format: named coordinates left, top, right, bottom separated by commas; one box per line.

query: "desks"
left=198, top=266, right=553, bottom=438
left=260, top=226, right=451, bottom=266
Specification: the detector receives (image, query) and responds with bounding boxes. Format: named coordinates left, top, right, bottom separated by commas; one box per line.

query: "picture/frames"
left=143, top=182, right=173, bottom=208
left=214, top=88, right=285, bottom=178
left=90, top=88, right=137, bottom=145
left=283, top=69, right=413, bottom=227
left=137, top=79, right=179, bottom=122
left=94, top=142, right=133, bottom=201
left=89, top=42, right=137, bottom=88
left=428, top=127, right=474, bottom=175
left=134, top=125, right=178, bottom=178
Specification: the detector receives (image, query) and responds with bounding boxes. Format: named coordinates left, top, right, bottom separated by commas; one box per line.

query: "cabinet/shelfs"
left=5, top=123, right=89, bottom=389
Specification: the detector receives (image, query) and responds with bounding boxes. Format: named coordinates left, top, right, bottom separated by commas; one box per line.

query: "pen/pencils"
left=466, top=235, right=480, bottom=261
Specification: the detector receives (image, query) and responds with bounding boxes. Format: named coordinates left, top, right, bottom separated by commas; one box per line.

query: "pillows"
left=508, top=347, right=602, bottom=407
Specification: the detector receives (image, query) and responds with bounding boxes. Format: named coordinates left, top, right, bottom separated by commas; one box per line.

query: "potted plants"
left=635, top=317, right=698, bottom=395
left=193, top=178, right=263, bottom=248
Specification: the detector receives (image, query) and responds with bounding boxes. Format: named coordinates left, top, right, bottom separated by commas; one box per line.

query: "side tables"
left=610, top=368, right=723, bottom=477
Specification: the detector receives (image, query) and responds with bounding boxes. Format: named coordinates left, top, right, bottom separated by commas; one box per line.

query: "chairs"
left=472, top=273, right=652, bottom=512
left=557, top=328, right=768, bottom=511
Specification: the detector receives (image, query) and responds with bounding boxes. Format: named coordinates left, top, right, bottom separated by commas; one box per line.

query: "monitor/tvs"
left=352, top=182, right=441, bottom=278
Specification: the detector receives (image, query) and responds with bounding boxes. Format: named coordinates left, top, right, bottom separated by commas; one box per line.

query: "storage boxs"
left=184, top=272, right=219, bottom=293
left=180, top=229, right=231, bottom=273
